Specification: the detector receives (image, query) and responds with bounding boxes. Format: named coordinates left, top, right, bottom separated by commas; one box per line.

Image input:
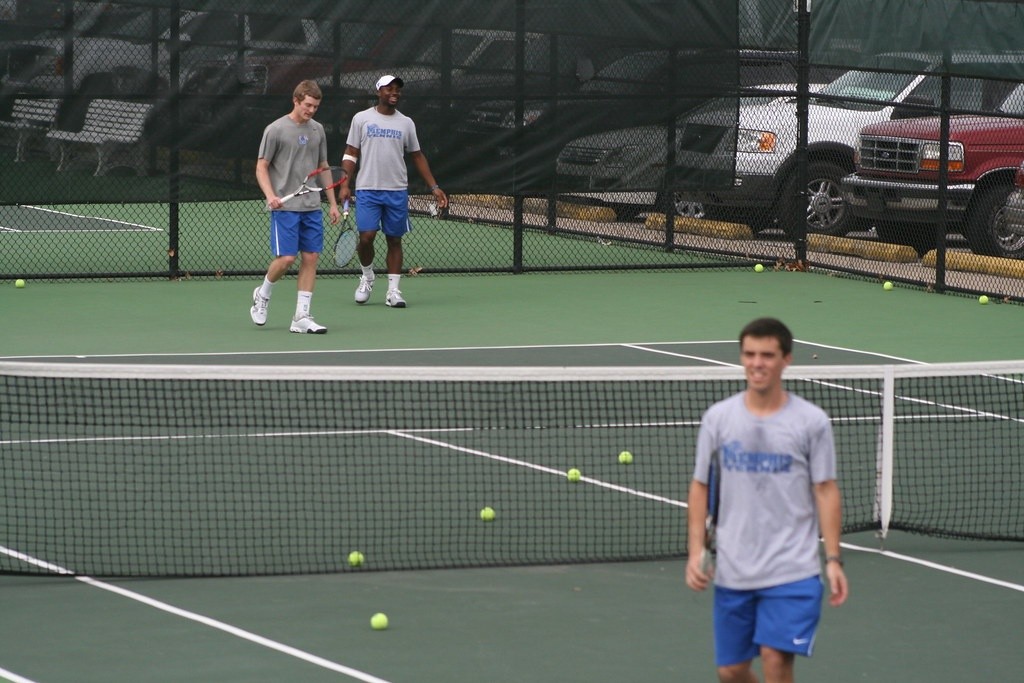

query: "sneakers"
left=355, top=270, right=375, bottom=303
left=250, top=286, right=270, bottom=325
left=290, top=315, right=327, bottom=334
left=386, top=287, right=406, bottom=307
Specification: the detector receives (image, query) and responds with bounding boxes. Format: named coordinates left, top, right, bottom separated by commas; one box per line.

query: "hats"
left=376, top=75, right=403, bottom=92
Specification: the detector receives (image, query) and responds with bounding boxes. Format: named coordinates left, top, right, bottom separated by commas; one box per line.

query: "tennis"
left=369, top=611, right=387, bottom=630
left=347, top=551, right=363, bottom=567
left=567, top=467, right=582, bottom=482
left=979, top=295, right=988, bottom=305
left=620, top=451, right=633, bottom=465
left=754, top=263, right=764, bottom=272
left=480, top=507, right=493, bottom=519
left=15, top=278, right=28, bottom=288
left=884, top=282, right=893, bottom=291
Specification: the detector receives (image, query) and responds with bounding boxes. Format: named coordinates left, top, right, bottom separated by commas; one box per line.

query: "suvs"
left=0, top=0, right=559, bottom=159
left=676, top=49, right=1024, bottom=238
left=454, top=49, right=831, bottom=191
left=839, top=82, right=1024, bottom=259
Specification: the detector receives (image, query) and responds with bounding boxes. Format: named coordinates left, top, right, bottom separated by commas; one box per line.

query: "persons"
left=251, top=80, right=341, bottom=334
left=339, top=73, right=448, bottom=309
left=685, top=317, right=849, bottom=682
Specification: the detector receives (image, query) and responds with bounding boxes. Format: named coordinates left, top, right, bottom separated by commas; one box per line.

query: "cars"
left=556, top=82, right=900, bottom=219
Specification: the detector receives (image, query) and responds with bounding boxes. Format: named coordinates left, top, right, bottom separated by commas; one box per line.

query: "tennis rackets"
left=266, top=166, right=350, bottom=212
left=333, top=197, right=360, bottom=269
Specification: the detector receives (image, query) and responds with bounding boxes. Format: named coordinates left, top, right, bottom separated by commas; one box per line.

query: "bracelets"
left=825, top=557, right=844, bottom=568
left=429, top=185, right=439, bottom=192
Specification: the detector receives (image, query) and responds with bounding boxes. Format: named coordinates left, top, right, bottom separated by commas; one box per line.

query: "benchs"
left=0, top=96, right=63, bottom=164
left=48, top=98, right=155, bottom=176
